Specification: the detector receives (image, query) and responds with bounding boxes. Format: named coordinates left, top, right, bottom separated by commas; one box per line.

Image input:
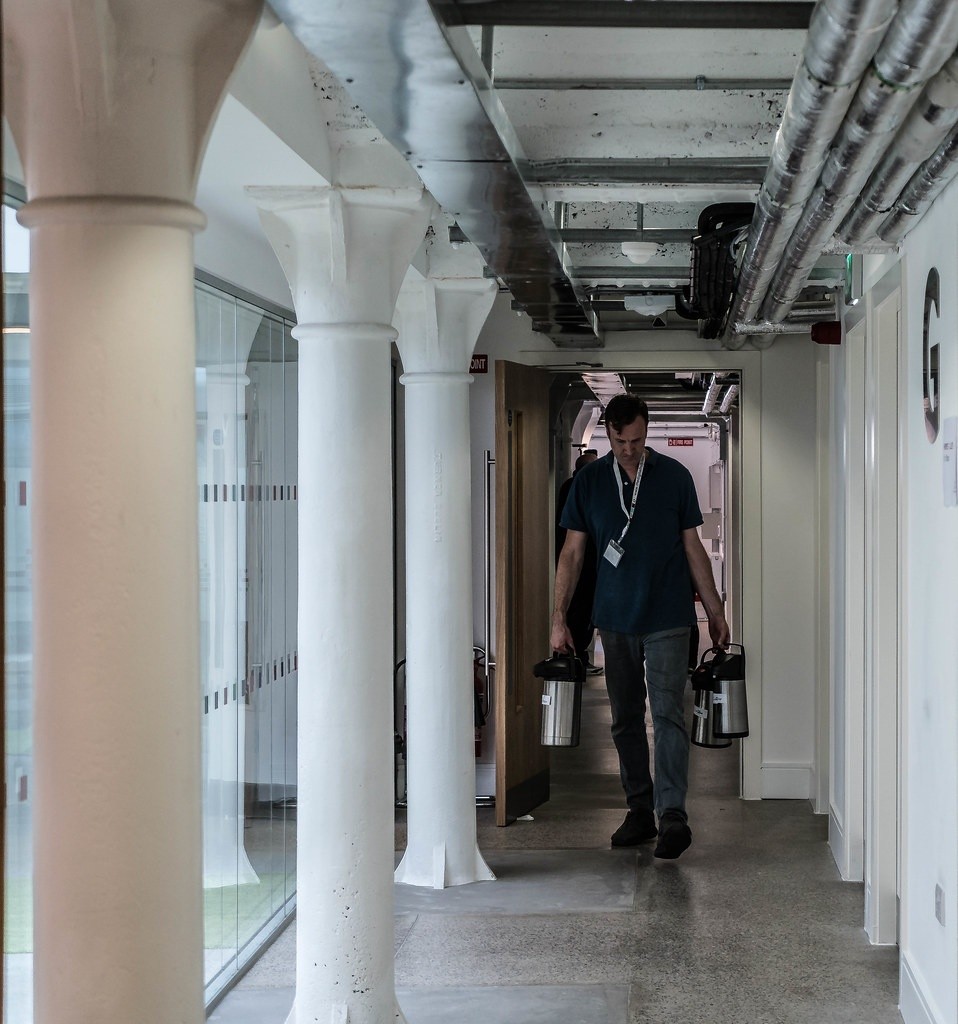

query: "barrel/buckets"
left=534, top=644, right=587, bottom=746
left=713, top=643, right=749, bottom=738
left=691, top=648, right=733, bottom=748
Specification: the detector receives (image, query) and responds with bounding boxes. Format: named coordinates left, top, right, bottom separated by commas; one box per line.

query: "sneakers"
left=653, top=821, right=692, bottom=859
left=611, top=810, right=658, bottom=849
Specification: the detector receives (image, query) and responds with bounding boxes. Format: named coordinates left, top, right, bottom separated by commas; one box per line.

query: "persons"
left=551, top=395, right=730, bottom=860
left=557, top=450, right=604, bottom=675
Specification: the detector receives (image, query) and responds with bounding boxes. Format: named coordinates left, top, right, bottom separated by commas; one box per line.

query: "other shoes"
left=587, top=662, right=604, bottom=675
